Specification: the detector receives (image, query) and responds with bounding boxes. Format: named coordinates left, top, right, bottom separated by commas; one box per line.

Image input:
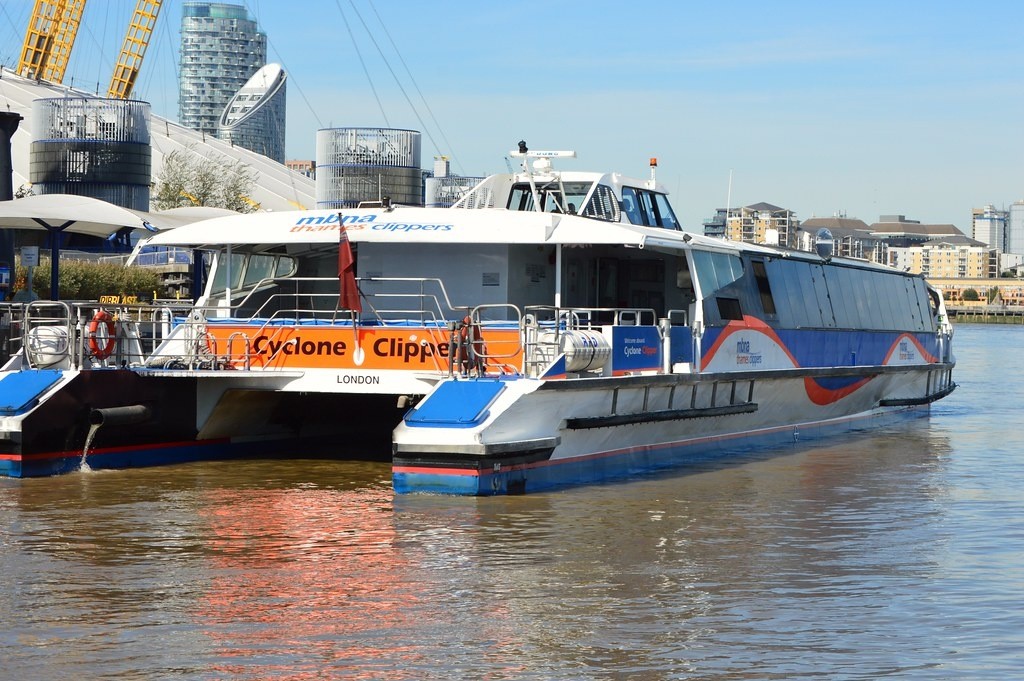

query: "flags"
left=337, top=216, right=364, bottom=314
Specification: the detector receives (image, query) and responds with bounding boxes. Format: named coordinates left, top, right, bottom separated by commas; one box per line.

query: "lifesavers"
left=460, top=317, right=481, bottom=368
left=90, top=312, right=115, bottom=360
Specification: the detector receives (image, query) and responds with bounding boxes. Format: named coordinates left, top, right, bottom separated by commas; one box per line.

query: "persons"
left=12, top=277, right=38, bottom=303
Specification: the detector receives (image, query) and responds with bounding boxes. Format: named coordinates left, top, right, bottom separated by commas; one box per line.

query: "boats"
left=1, top=138, right=960, bottom=498
